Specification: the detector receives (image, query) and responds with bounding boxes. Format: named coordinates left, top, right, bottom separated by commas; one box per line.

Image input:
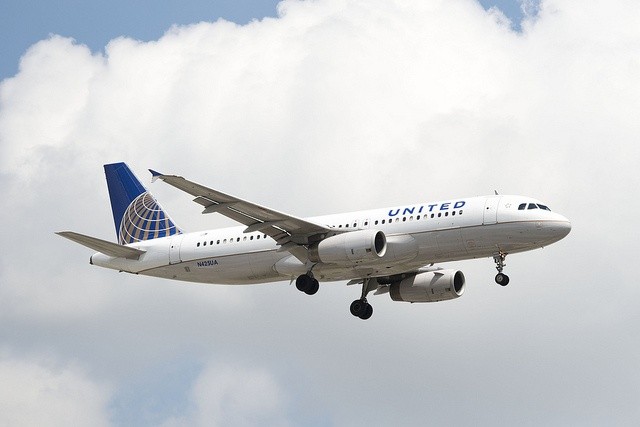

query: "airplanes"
left=55, top=160, right=573, bottom=321
